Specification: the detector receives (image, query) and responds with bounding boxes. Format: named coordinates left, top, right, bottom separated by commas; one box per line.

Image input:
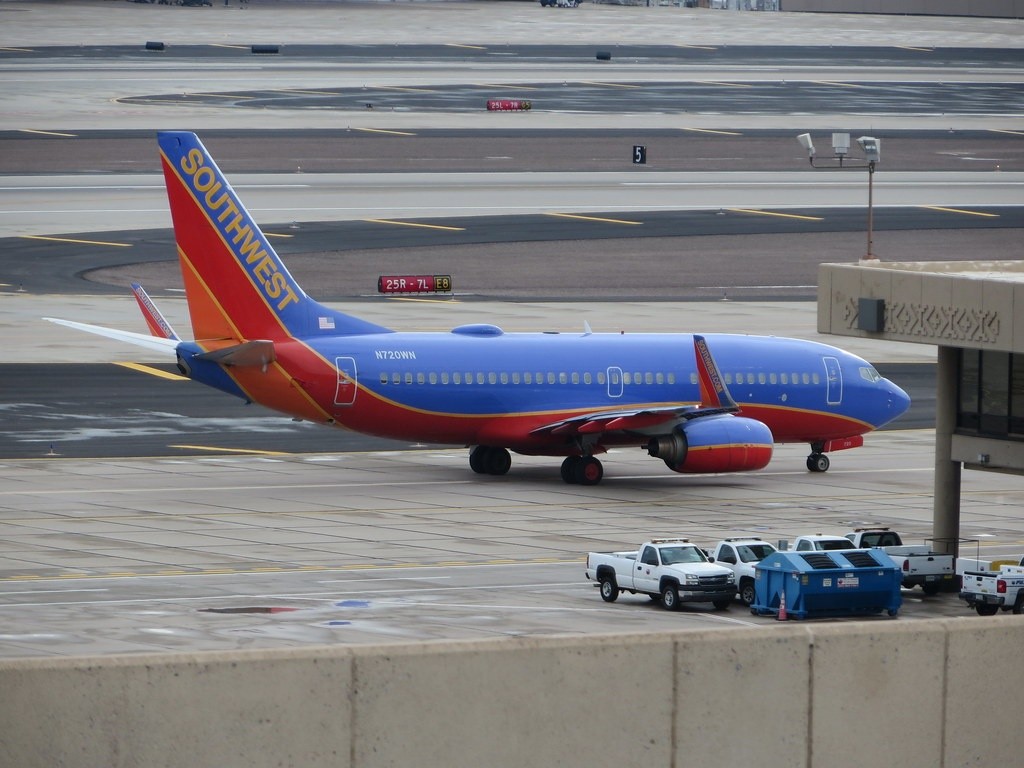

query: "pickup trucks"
left=958, top=556, right=1024, bottom=617
left=701, top=536, right=779, bottom=608
left=787, top=532, right=857, bottom=552
left=585, top=537, right=738, bottom=612
left=843, top=526, right=956, bottom=595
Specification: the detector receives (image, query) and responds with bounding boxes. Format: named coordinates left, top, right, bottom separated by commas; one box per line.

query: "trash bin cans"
left=749, top=547, right=903, bottom=620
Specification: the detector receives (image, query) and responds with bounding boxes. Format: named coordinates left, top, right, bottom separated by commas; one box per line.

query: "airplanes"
left=39, top=131, right=910, bottom=488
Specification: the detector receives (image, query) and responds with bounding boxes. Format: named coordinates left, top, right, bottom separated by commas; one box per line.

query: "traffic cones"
left=775, top=591, right=789, bottom=621
